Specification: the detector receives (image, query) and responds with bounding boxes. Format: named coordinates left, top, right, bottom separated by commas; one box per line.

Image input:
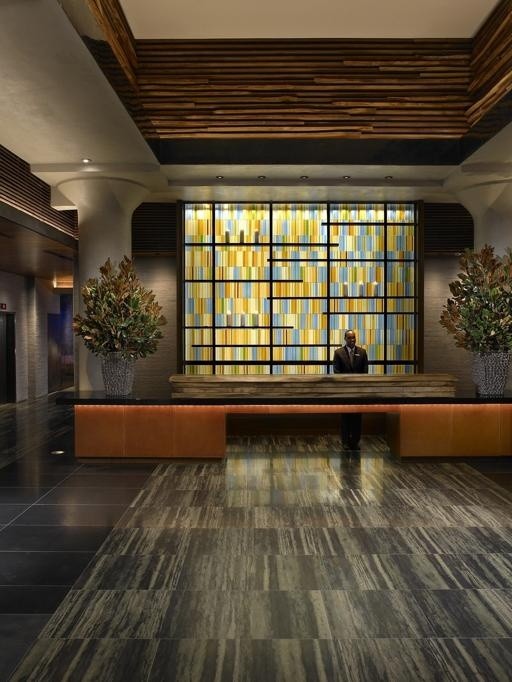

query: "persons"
left=332, top=329, right=368, bottom=450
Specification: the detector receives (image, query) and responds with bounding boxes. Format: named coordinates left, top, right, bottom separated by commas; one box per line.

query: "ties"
left=350, top=349, right=354, bottom=369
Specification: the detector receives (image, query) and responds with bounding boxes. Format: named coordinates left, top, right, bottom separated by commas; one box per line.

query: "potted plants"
left=440, top=244, right=512, bottom=395
left=73, top=255, right=167, bottom=397
